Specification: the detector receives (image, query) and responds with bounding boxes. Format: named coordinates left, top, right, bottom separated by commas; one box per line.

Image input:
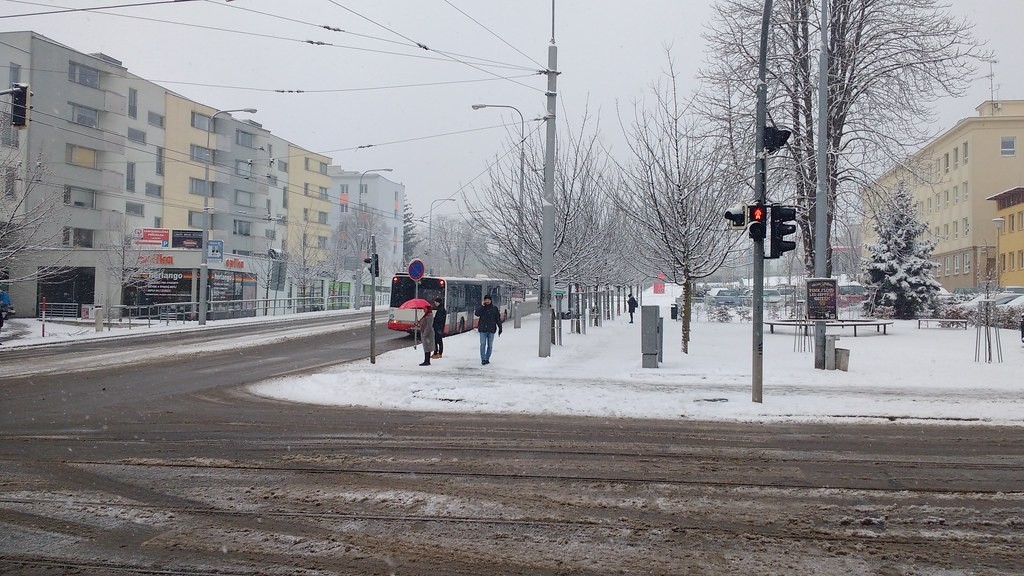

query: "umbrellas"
left=400, top=298, right=430, bottom=326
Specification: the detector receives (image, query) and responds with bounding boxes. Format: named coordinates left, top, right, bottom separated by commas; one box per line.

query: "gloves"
left=499, top=327, right=502, bottom=335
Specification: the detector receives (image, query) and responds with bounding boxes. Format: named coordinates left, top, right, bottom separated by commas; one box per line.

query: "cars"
left=935, top=285, right=1024, bottom=330
left=704, top=280, right=866, bottom=310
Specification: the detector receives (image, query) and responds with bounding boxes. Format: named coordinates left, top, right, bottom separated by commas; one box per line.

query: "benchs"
left=764, top=318, right=894, bottom=337
left=918, top=319, right=968, bottom=329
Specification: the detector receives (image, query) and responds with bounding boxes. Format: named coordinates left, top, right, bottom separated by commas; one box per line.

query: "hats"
left=484, top=295, right=491, bottom=300
left=434, top=298, right=442, bottom=303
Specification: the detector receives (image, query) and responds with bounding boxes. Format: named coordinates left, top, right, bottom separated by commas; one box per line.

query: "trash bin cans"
left=670, top=303, right=678, bottom=320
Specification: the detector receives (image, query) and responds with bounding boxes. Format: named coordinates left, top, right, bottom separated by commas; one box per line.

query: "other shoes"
left=430, top=354, right=442, bottom=358
left=482, top=360, right=490, bottom=365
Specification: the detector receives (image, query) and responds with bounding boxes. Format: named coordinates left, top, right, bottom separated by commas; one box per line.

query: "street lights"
left=354, top=168, right=393, bottom=311
left=427, top=197, right=456, bottom=277
left=471, top=103, right=526, bottom=329
left=197, top=107, right=257, bottom=326
left=991, top=218, right=1006, bottom=291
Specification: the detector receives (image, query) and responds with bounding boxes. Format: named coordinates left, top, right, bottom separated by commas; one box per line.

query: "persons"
left=417, top=306, right=435, bottom=366
left=475, top=295, right=502, bottom=365
left=627, top=293, right=636, bottom=323
left=0, top=289, right=10, bottom=320
left=431, top=298, right=446, bottom=359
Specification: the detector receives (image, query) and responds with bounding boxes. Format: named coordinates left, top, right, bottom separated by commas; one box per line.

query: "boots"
left=419, top=352, right=430, bottom=366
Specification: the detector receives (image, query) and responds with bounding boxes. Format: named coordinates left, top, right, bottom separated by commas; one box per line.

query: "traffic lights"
left=748, top=205, right=767, bottom=240
left=724, top=204, right=748, bottom=230
left=770, top=205, right=797, bottom=257
left=766, top=127, right=792, bottom=152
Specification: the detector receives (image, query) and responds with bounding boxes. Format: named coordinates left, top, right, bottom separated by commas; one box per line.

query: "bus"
left=386, top=272, right=525, bottom=338
